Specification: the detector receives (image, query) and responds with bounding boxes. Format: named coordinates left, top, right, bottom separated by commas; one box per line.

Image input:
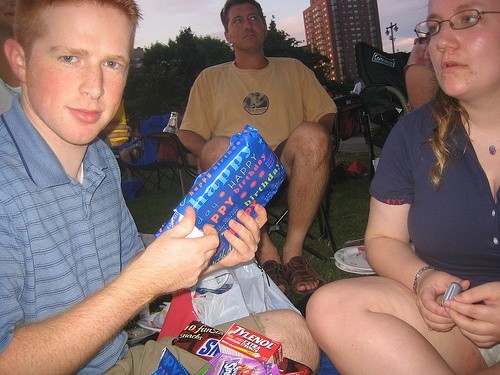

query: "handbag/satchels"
left=191, top=259, right=301, bottom=327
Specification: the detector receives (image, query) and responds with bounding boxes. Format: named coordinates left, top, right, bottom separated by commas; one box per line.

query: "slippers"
left=262, top=260, right=291, bottom=297
left=280, top=256, right=324, bottom=294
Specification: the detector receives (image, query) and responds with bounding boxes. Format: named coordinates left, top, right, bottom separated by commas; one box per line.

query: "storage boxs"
left=172, top=320, right=283, bottom=375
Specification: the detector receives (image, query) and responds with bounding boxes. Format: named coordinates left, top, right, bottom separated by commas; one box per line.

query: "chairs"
left=110, top=113, right=182, bottom=191
left=149, top=104, right=362, bottom=261
left=354, top=41, right=410, bottom=178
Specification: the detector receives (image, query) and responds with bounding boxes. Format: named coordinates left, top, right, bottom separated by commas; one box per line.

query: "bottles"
left=334, top=246, right=377, bottom=275
left=163, top=111, right=178, bottom=134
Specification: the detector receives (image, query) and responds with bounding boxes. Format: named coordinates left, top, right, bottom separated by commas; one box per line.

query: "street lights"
left=385, top=22, right=398, bottom=54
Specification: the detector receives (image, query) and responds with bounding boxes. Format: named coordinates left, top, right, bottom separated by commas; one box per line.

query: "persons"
left=0, top=0, right=321, bottom=375
left=305, top=0, right=500, bottom=375
left=350, top=73, right=361, bottom=97
left=176, top=0, right=337, bottom=295
left=405, top=36, right=440, bottom=111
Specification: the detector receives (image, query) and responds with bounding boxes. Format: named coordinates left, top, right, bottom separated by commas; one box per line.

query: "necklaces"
left=489, top=142, right=497, bottom=156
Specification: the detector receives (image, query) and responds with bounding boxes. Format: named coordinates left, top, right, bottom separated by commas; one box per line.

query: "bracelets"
left=413, top=266, right=437, bottom=295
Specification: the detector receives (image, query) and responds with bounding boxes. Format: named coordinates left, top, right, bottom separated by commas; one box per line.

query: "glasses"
left=414, top=9, right=500, bottom=39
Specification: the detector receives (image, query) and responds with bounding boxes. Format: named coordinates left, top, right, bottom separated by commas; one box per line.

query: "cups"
left=104, top=98, right=129, bottom=142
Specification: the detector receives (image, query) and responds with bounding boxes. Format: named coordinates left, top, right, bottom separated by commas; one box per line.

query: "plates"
left=127, top=330, right=156, bottom=343
left=138, top=312, right=162, bottom=332
